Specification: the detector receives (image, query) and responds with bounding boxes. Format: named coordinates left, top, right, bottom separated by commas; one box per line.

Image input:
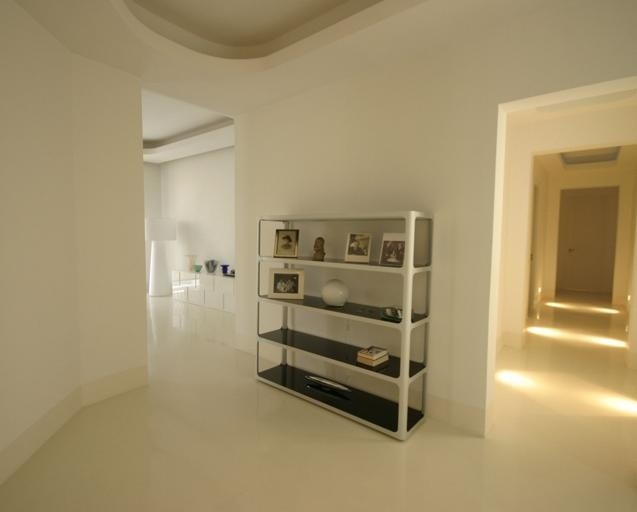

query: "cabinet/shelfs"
left=170, top=269, right=235, bottom=314
left=254, top=210, right=435, bottom=441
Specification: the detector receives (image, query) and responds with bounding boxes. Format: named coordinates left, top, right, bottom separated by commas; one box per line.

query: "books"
left=356, top=352, right=389, bottom=366
left=356, top=345, right=388, bottom=359
left=357, top=358, right=392, bottom=371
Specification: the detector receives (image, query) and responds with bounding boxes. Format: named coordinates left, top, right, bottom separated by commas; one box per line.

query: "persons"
left=275, top=278, right=296, bottom=292
left=349, top=237, right=404, bottom=264
left=280, top=235, right=293, bottom=249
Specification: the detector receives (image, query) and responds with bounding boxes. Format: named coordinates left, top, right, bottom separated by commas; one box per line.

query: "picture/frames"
left=267, top=267, right=304, bottom=300
left=378, top=232, right=405, bottom=267
left=343, top=232, right=371, bottom=263
left=273, top=229, right=300, bottom=258
